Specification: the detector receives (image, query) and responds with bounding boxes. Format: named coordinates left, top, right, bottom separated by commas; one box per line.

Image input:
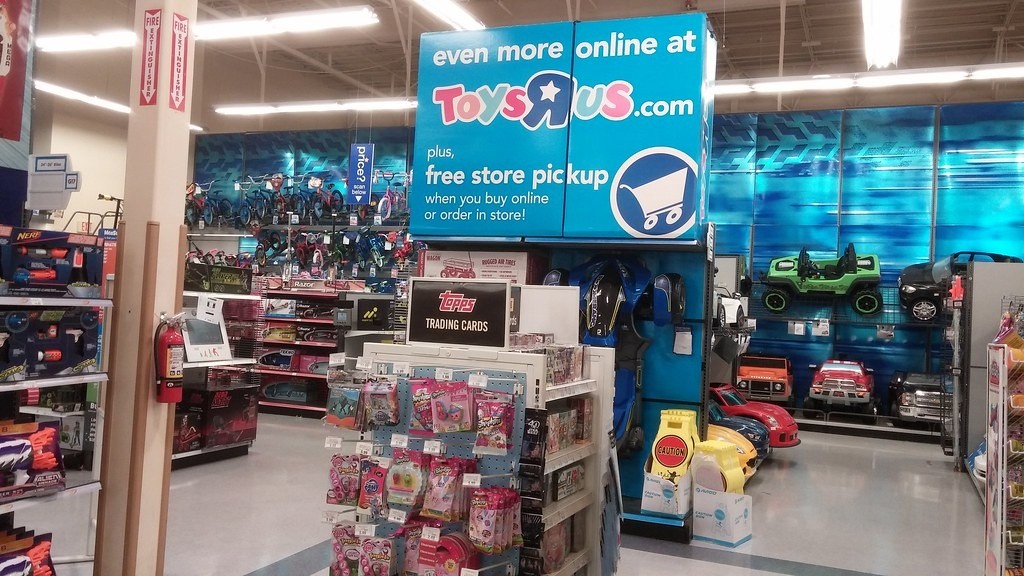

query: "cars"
left=712, top=285, right=745, bottom=329
left=709, top=384, right=801, bottom=481
left=898, top=251, right=1023, bottom=324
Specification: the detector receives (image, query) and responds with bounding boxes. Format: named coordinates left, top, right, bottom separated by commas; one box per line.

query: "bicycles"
left=185, top=169, right=417, bottom=272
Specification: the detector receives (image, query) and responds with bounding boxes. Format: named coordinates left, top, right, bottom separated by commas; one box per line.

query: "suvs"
left=802, top=359, right=877, bottom=423
left=758, top=242, right=883, bottom=317
left=736, top=355, right=795, bottom=415
left=890, top=370, right=942, bottom=428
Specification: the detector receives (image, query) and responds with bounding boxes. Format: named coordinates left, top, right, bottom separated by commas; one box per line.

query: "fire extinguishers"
left=152, top=311, right=188, bottom=404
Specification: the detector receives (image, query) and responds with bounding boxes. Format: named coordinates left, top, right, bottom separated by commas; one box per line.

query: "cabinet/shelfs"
left=0, top=292, right=106, bottom=527
left=252, top=272, right=395, bottom=414
left=975, top=322, right=1023, bottom=576
left=710, top=287, right=959, bottom=458
left=169, top=287, right=256, bottom=460
left=328, top=336, right=596, bottom=576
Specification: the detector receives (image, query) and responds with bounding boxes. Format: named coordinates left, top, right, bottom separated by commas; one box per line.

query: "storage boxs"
left=169, top=249, right=259, bottom=464
left=0, top=215, right=109, bottom=576
left=256, top=273, right=366, bottom=406
